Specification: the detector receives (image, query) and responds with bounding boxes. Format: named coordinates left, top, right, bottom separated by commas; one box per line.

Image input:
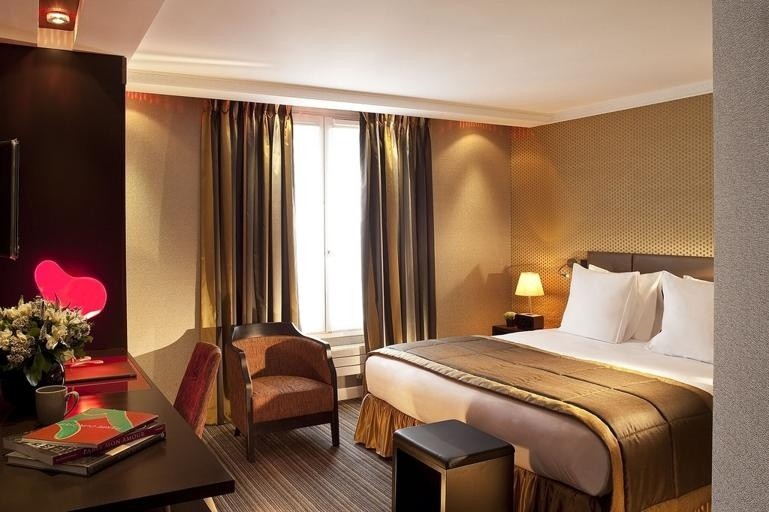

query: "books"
left=3, top=420, right=167, bottom=466
left=6, top=432, right=167, bottom=476
left=64, top=361, right=137, bottom=384
left=22, top=407, right=159, bottom=448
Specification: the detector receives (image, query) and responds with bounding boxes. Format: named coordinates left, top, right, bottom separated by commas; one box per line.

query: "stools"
left=389, top=419, right=515, bottom=512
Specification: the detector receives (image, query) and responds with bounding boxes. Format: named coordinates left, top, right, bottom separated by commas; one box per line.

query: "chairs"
left=224, top=321, right=340, bottom=464
left=160, top=341, right=222, bottom=512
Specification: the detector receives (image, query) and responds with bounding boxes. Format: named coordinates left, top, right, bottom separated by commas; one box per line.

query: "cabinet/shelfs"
left=0, top=43, right=127, bottom=354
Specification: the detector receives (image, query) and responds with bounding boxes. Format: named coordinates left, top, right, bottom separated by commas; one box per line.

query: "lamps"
left=514, top=271, right=544, bottom=314
left=557, top=258, right=577, bottom=278
left=36, top=0, right=80, bottom=51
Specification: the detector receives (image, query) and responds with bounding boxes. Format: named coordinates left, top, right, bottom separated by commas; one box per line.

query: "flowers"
left=0, top=295, right=95, bottom=386
left=503, top=311, right=517, bottom=320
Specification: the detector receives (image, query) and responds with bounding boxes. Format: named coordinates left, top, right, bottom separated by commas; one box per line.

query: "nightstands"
left=491, top=325, right=540, bottom=335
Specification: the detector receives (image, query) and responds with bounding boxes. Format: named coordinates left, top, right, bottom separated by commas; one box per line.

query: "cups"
left=36, top=385, right=79, bottom=423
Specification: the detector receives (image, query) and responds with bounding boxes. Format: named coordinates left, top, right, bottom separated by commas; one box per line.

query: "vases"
left=506, top=320, right=516, bottom=327
left=0, top=351, right=65, bottom=420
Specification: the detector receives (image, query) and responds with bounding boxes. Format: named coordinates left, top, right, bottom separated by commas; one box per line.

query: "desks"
left=0, top=356, right=234, bottom=512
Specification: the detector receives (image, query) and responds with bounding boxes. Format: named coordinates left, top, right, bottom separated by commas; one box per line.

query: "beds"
left=352, top=250, right=714, bottom=512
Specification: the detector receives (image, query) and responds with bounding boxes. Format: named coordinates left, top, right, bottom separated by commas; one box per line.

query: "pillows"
left=558, top=262, right=714, bottom=365
